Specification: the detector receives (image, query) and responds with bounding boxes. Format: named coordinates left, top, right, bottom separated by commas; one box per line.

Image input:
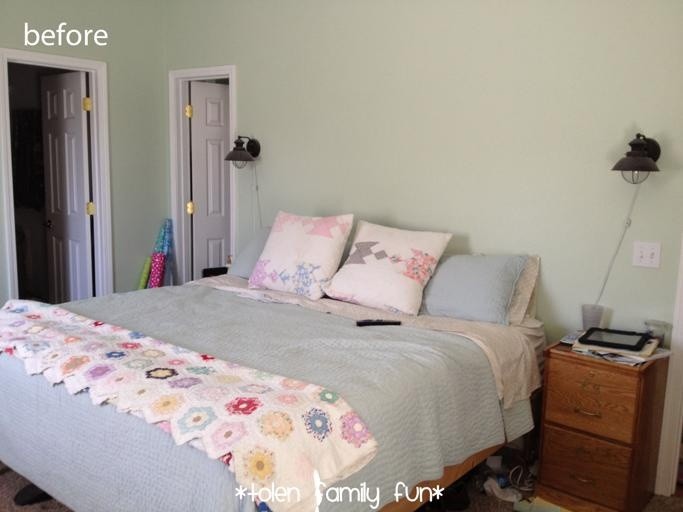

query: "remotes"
left=356, top=319, right=402, bottom=326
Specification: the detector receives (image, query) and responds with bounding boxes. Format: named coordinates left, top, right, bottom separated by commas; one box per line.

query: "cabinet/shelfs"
left=536, top=339, right=658, bottom=512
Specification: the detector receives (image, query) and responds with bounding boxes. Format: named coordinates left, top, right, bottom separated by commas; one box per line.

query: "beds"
left=1, top=274, right=545, bottom=512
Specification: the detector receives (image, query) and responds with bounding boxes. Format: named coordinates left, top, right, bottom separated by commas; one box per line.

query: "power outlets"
left=632, top=242, right=661, bottom=268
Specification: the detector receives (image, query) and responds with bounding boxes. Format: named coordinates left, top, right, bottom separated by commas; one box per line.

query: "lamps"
left=610, top=131, right=662, bottom=185
left=224, top=134, right=261, bottom=171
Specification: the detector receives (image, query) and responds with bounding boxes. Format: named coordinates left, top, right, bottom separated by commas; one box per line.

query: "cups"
left=645, top=320, right=665, bottom=348
left=582, top=304, right=603, bottom=332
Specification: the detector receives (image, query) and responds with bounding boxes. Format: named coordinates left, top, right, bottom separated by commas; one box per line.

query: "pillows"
left=249, top=210, right=354, bottom=301
left=228, top=225, right=271, bottom=286
left=508, top=255, right=540, bottom=327
left=318, top=220, right=453, bottom=317
left=418, top=251, right=528, bottom=327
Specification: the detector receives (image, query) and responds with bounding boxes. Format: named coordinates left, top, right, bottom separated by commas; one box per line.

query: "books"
left=572, top=326, right=677, bottom=368
